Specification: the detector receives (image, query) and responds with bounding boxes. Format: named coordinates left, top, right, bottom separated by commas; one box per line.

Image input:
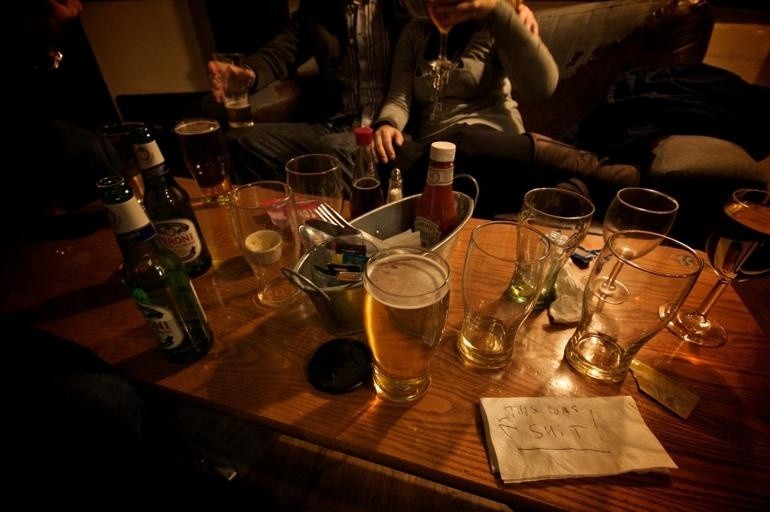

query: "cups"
left=174, top=117, right=235, bottom=208
left=459, top=217, right=549, bottom=374
left=281, top=149, right=345, bottom=227
left=211, top=51, right=256, bottom=130
left=509, top=184, right=595, bottom=309
left=243, top=226, right=284, bottom=264
left=228, top=179, right=304, bottom=311
left=361, top=248, right=452, bottom=407
left=566, top=229, right=703, bottom=390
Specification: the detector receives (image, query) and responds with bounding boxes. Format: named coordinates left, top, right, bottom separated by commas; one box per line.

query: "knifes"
left=303, top=219, right=354, bottom=238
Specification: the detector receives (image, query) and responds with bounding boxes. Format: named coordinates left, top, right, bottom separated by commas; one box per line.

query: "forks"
left=315, top=200, right=398, bottom=256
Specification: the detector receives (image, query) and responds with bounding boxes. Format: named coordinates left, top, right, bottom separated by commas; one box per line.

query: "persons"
left=207, top=0, right=414, bottom=200
left=365, top=0, right=642, bottom=205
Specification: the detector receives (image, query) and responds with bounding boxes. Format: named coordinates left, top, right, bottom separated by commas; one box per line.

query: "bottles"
left=98, top=174, right=217, bottom=362
left=349, top=128, right=380, bottom=213
left=128, top=124, right=213, bottom=282
left=413, top=141, right=460, bottom=246
left=384, top=162, right=405, bottom=206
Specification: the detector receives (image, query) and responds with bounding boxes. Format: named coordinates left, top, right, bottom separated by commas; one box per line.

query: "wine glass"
left=662, top=188, right=770, bottom=349
left=589, top=181, right=679, bottom=308
left=427, top=0, right=459, bottom=67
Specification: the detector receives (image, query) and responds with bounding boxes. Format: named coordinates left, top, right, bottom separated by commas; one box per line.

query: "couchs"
left=245, top=1, right=760, bottom=203
